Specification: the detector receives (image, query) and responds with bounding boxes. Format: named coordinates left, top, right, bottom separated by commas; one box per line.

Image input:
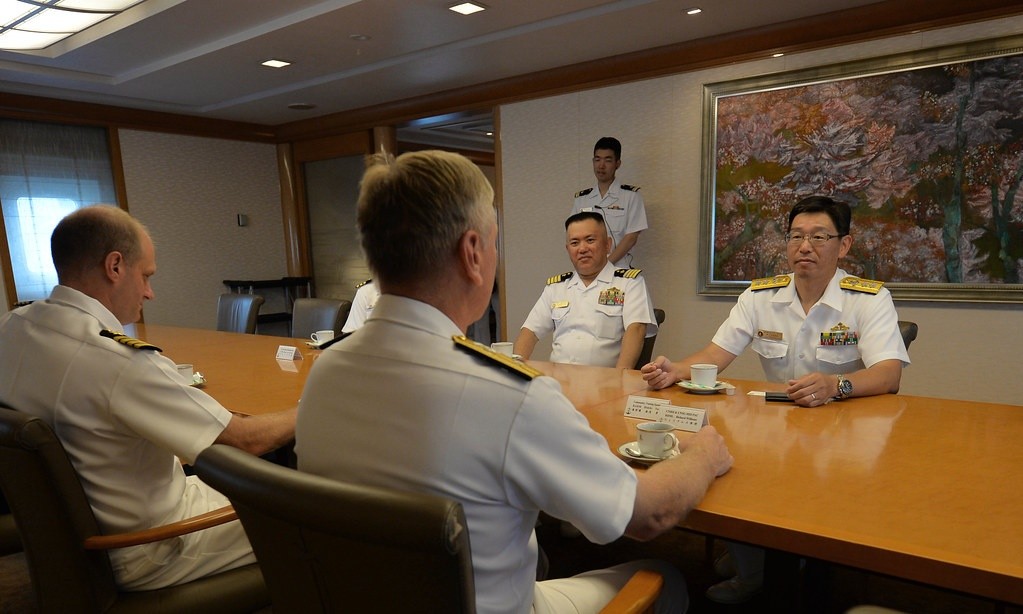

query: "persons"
left=570, top=137, right=648, bottom=269
left=641, top=195, right=912, bottom=408
left=0, top=204, right=299, bottom=590
left=513, top=211, right=658, bottom=369
left=294, top=141, right=733, bottom=614
left=342, top=278, right=379, bottom=334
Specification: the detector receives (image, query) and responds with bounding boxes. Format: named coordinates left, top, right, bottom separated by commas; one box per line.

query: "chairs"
left=292, top=298, right=352, bottom=339
left=634, top=309, right=665, bottom=371
left=194, top=444, right=665, bottom=614
left=0, top=407, right=271, bottom=614
left=215, top=293, right=265, bottom=334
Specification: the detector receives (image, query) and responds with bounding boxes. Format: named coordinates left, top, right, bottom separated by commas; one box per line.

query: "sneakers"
left=713, top=544, right=764, bottom=576
left=705, top=569, right=773, bottom=604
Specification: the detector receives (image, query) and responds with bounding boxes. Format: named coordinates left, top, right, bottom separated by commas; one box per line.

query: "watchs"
left=833, top=374, right=853, bottom=400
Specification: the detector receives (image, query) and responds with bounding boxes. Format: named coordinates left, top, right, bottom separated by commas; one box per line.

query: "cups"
left=311, top=330, right=334, bottom=344
left=177, top=364, right=194, bottom=384
left=491, top=342, right=513, bottom=358
left=636, top=422, right=677, bottom=458
left=691, top=363, right=718, bottom=388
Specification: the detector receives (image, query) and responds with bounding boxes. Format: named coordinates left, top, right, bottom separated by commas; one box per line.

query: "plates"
left=617, top=441, right=679, bottom=466
left=305, top=342, right=321, bottom=349
left=676, top=380, right=735, bottom=394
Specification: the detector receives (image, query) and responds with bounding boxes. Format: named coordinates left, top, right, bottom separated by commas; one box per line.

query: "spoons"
left=625, top=448, right=666, bottom=460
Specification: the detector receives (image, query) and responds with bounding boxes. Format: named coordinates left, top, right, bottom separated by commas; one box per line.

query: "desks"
left=119, top=320, right=1023, bottom=614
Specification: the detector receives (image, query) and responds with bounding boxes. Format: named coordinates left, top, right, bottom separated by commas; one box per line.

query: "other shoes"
left=560, top=519, right=582, bottom=539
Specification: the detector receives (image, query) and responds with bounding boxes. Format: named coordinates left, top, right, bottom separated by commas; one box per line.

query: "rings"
left=811, top=394, right=816, bottom=399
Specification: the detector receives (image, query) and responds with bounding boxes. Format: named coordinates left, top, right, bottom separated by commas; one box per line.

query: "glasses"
left=785, top=232, right=845, bottom=246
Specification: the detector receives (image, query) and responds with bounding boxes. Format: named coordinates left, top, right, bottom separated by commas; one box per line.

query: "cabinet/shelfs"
left=223, top=275, right=312, bottom=338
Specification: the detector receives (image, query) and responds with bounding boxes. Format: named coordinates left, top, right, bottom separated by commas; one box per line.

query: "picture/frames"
left=694, top=33, right=1023, bottom=304
